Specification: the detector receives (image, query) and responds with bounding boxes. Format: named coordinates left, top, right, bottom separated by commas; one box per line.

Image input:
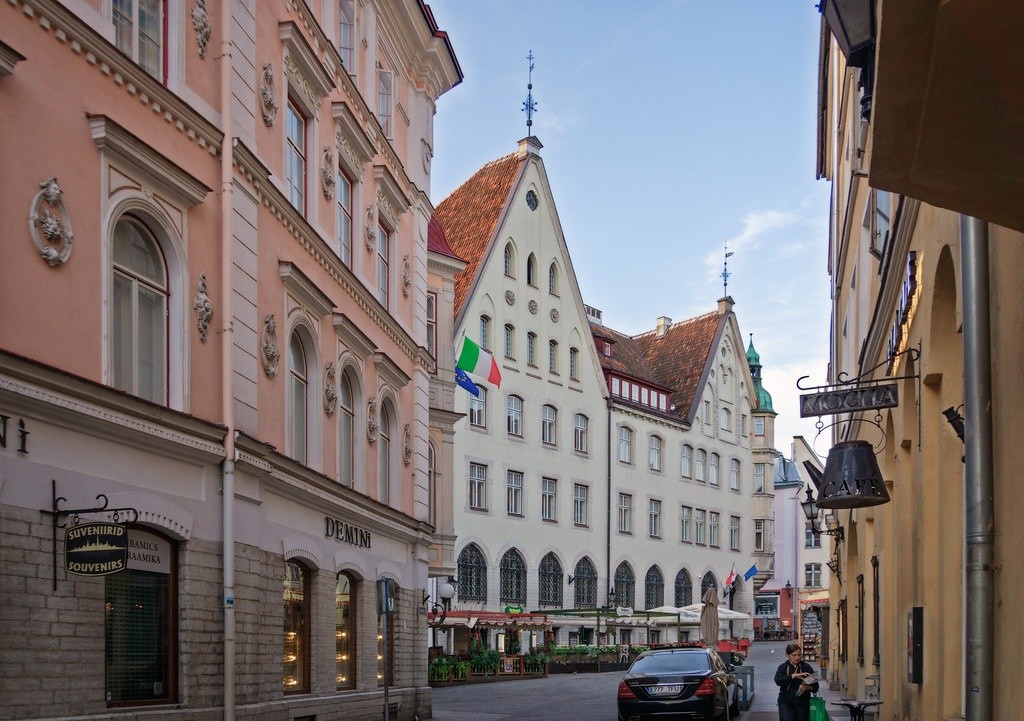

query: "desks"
left=831, top=699, right=883, bottom=721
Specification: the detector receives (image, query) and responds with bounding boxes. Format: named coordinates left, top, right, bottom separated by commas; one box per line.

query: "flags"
left=454, top=359, right=479, bottom=398
left=725, top=567, right=736, bottom=584
left=454, top=335, right=501, bottom=389
left=744, top=564, right=757, bottom=582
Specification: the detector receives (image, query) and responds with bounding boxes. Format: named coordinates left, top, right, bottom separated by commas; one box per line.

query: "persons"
left=773, top=644, right=819, bottom=721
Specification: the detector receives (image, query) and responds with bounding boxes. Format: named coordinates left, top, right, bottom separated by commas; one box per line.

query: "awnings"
left=645, top=603, right=750, bottom=643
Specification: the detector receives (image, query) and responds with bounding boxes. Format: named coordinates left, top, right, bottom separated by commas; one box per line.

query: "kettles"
left=800, top=418, right=894, bottom=510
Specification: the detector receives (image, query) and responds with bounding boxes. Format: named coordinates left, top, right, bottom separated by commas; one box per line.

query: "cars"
left=617, top=648, right=740, bottom=721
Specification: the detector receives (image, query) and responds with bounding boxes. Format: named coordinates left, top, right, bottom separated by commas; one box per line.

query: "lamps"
left=422, top=582, right=455, bottom=629
left=601, top=586, right=616, bottom=613
left=800, top=482, right=845, bottom=543
left=815, top=0, right=877, bottom=122
left=785, top=580, right=792, bottom=598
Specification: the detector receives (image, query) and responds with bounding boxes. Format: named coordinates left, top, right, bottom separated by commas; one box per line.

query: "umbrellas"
left=700, top=584, right=719, bottom=644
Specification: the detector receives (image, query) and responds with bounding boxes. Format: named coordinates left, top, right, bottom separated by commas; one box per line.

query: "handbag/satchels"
left=809, top=689, right=830, bottom=721
